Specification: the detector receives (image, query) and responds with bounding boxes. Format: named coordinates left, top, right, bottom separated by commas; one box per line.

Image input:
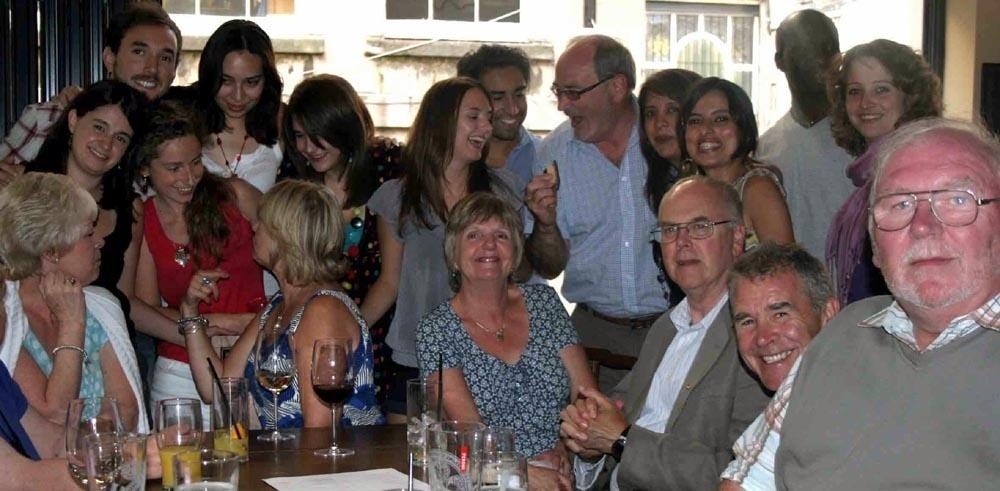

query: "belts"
left=577, top=302, right=657, bottom=329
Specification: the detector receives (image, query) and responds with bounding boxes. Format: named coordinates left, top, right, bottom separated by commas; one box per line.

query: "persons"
left=1, top=0, right=999, bottom=490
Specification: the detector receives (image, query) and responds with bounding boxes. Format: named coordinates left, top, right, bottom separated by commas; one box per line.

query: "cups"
left=406, top=377, right=529, bottom=491
left=65, top=376, right=250, bottom=490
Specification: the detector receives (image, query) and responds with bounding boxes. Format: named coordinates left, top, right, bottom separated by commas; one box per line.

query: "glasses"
left=650, top=218, right=732, bottom=244
left=868, top=188, right=1000, bottom=232
left=550, top=71, right=614, bottom=100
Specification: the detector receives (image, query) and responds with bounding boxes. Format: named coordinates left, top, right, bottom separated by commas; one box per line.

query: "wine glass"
left=309, top=336, right=355, bottom=457
left=255, top=332, right=298, bottom=440
